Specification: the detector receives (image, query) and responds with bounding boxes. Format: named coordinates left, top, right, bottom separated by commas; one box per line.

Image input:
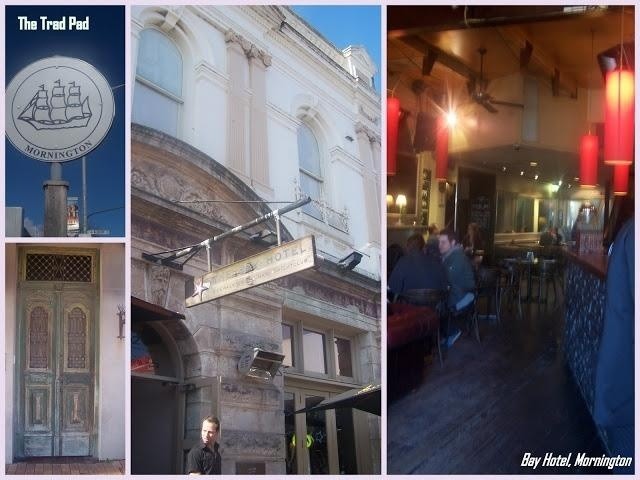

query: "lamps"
left=578, top=5, right=599, bottom=186
left=251, top=231, right=286, bottom=248
left=388, top=35, right=400, bottom=176
left=612, top=6, right=631, bottom=198
left=603, top=6, right=635, bottom=165
left=435, top=61, right=449, bottom=182
left=341, top=253, right=363, bottom=271
left=237, top=347, right=285, bottom=385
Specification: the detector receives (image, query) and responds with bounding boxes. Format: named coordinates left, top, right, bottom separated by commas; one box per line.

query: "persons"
left=187, top=416, right=221, bottom=475
left=591, top=162, right=635, bottom=474
left=390, top=229, right=476, bottom=346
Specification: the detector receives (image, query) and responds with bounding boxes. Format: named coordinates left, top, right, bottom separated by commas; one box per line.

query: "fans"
left=447, top=47, right=525, bottom=115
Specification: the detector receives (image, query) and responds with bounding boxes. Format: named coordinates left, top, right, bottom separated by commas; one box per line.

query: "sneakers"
left=441, top=329, right=461, bottom=347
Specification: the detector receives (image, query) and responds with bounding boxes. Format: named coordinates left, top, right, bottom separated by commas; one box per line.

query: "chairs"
left=386, top=241, right=563, bottom=374
